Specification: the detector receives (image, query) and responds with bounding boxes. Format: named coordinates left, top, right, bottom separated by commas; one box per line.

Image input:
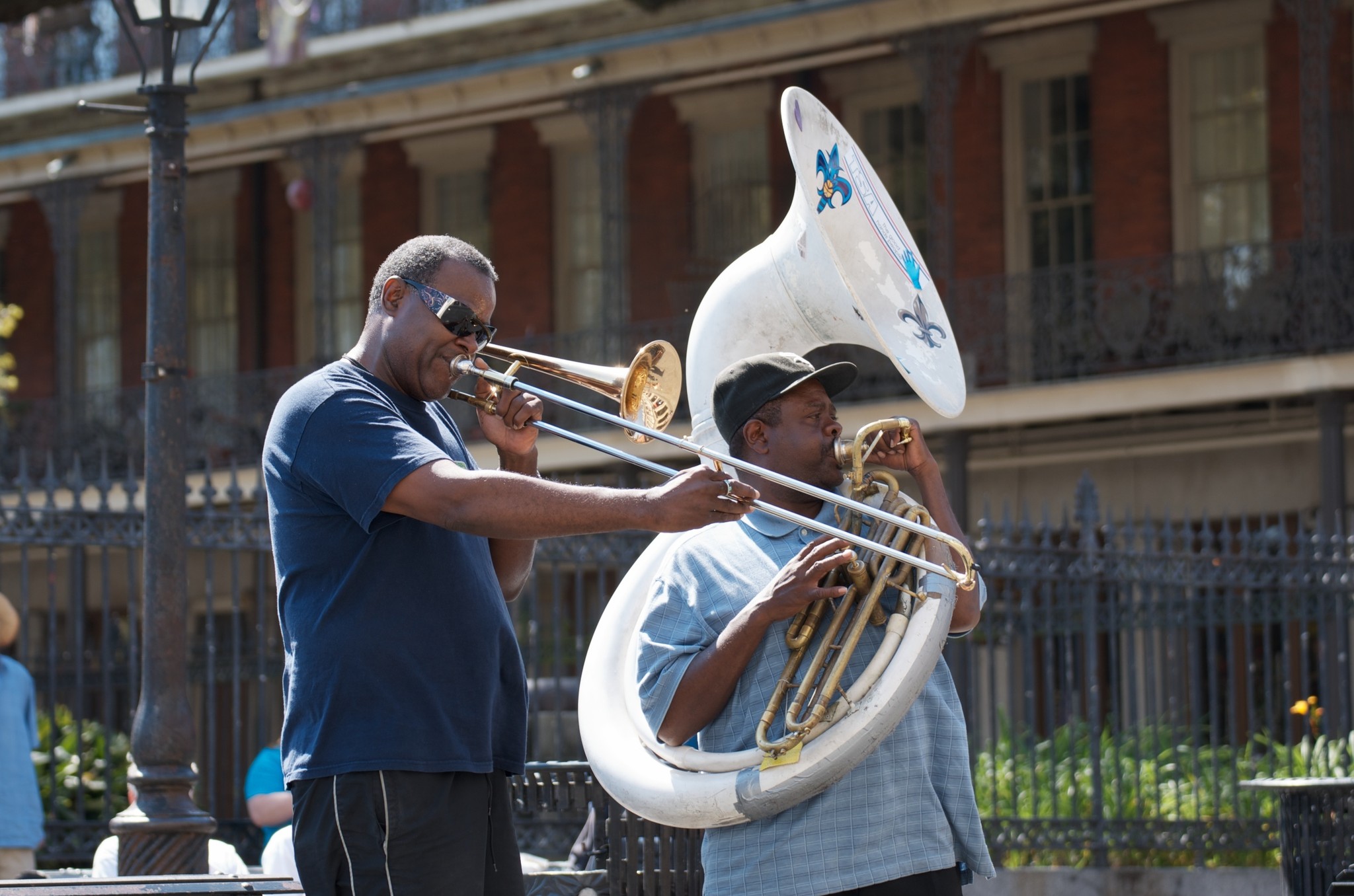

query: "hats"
left=712, top=350, right=859, bottom=443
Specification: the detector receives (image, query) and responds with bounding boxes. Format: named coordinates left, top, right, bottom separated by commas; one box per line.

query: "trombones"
left=446, top=339, right=981, bottom=593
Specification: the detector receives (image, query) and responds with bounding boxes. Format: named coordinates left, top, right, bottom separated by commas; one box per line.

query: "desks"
left=1237, top=777, right=1354, bottom=896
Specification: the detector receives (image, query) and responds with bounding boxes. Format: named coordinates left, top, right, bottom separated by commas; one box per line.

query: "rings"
left=722, top=479, right=732, bottom=496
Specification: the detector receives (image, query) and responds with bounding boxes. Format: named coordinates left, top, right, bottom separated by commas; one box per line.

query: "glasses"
left=402, top=277, right=496, bottom=348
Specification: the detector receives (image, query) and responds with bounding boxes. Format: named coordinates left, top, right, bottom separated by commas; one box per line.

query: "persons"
left=637, top=352, right=997, bottom=896
left=244, top=738, right=293, bottom=868
left=262, top=237, right=761, bottom=896
left=566, top=733, right=698, bottom=872
left=0, top=594, right=44, bottom=879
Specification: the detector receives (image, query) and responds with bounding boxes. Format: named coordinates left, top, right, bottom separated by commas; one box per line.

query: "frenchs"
left=576, top=86, right=966, bottom=830
